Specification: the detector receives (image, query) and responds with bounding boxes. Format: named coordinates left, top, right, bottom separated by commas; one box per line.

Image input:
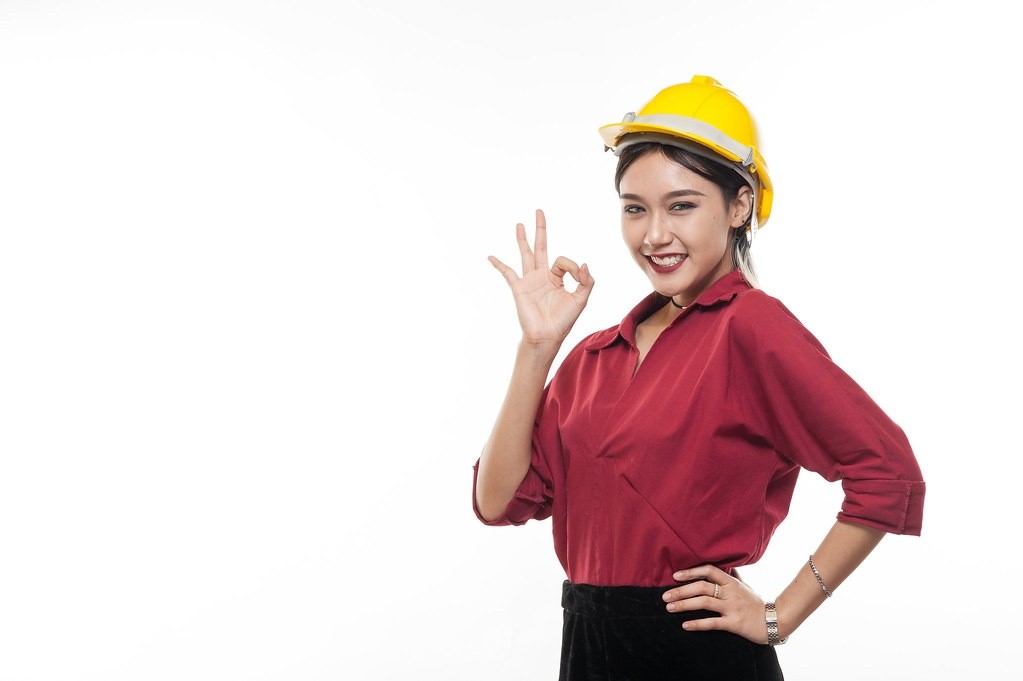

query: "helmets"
left=599, top=74, right=774, bottom=234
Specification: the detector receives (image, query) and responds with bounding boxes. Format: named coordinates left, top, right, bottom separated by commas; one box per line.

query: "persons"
left=471, top=74, right=927, bottom=680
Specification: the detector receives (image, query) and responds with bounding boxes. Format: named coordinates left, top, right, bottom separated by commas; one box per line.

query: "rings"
left=713, top=584, right=719, bottom=598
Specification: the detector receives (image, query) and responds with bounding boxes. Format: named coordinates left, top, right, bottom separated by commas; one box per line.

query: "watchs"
left=765, top=602, right=789, bottom=645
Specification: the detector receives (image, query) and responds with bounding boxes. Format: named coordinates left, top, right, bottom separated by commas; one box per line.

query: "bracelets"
left=809, top=555, right=832, bottom=598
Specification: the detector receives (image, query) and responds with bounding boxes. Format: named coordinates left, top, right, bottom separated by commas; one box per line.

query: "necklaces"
left=670, top=296, right=687, bottom=310
